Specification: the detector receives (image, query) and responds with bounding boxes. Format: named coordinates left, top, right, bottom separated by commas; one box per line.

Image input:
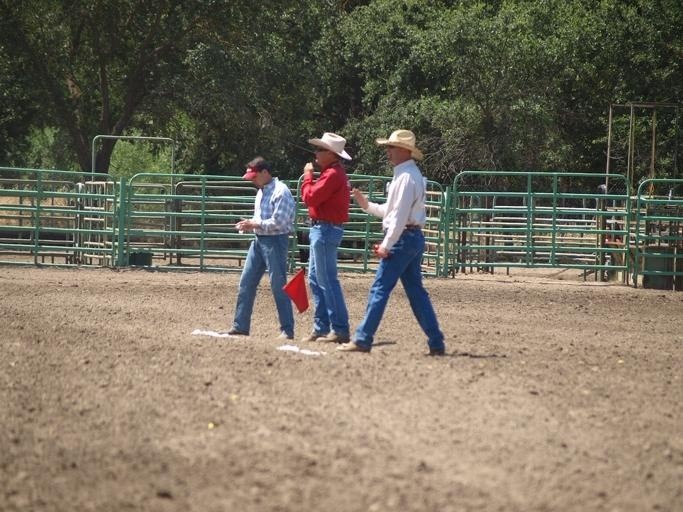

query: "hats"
left=243, top=163, right=270, bottom=180
left=308, top=133, right=353, bottom=161
left=376, top=128, right=423, bottom=161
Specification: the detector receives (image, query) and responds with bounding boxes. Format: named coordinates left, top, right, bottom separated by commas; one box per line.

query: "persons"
left=225, top=156, right=296, bottom=338
left=300, top=133, right=353, bottom=344
left=336, top=129, right=447, bottom=355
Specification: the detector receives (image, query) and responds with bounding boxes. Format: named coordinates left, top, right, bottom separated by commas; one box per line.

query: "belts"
left=308, top=218, right=329, bottom=225
left=383, top=225, right=420, bottom=236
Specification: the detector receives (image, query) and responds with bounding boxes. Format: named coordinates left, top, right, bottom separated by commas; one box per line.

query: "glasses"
left=315, top=145, right=327, bottom=151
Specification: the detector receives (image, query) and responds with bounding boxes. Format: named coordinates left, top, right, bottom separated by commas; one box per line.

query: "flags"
left=281, top=267, right=310, bottom=312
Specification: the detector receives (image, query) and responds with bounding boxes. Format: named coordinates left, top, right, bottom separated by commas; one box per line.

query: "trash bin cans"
left=642, top=246, right=683, bottom=289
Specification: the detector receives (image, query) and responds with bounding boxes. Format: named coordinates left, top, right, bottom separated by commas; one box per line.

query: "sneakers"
left=423, top=349, right=445, bottom=355
left=301, top=333, right=369, bottom=352
left=276, top=331, right=293, bottom=340
left=218, top=329, right=244, bottom=335
